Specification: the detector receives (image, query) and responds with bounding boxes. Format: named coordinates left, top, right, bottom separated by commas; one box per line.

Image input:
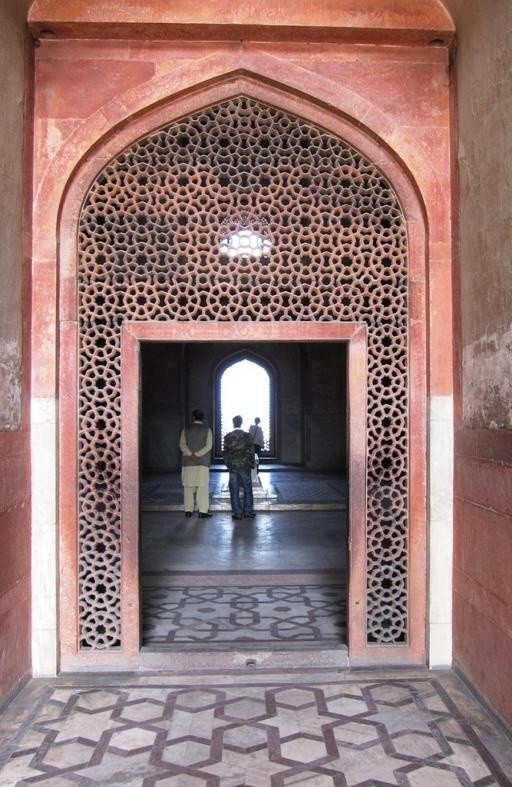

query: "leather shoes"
left=186, top=511, right=192, bottom=517
left=199, top=512, right=212, bottom=517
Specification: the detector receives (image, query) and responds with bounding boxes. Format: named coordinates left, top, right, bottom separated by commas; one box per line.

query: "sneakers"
left=244, top=513, right=255, bottom=519
left=232, top=514, right=242, bottom=520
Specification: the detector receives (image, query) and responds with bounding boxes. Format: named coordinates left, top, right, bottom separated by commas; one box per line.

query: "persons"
left=249, top=414, right=264, bottom=475
left=179, top=407, right=215, bottom=517
left=222, top=414, right=257, bottom=521
